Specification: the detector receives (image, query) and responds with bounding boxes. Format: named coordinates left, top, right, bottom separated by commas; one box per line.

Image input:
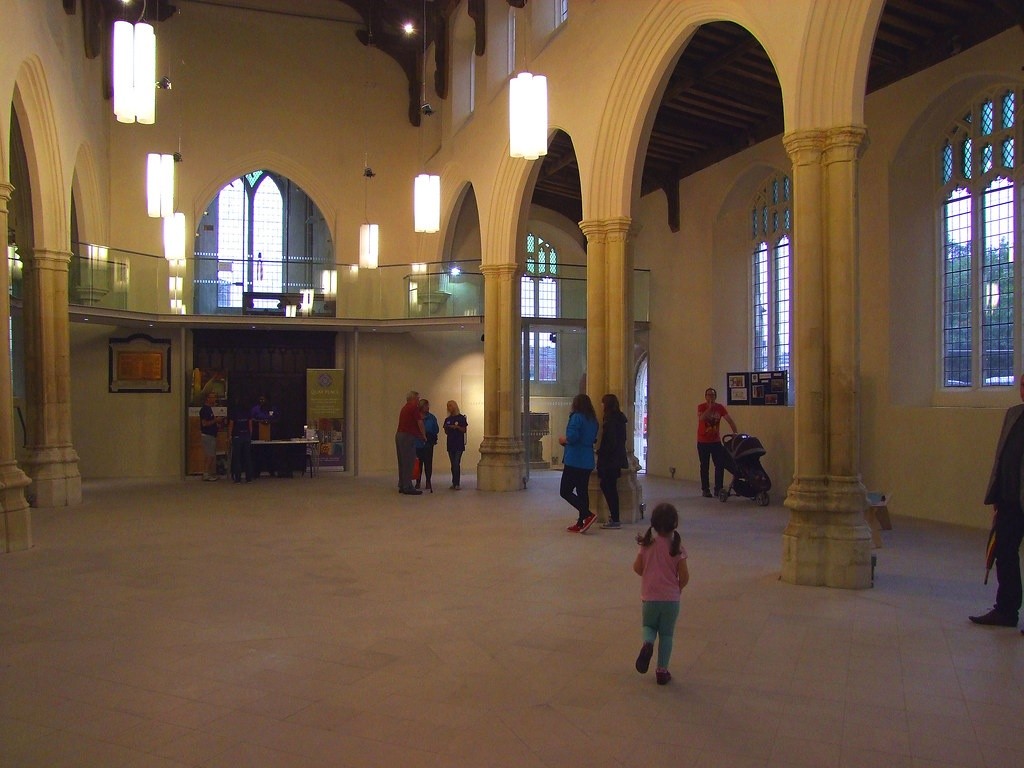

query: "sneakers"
left=580, top=513, right=597, bottom=533
left=567, top=520, right=582, bottom=532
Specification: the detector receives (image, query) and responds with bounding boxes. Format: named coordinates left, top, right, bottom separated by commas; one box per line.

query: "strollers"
left=718, top=432, right=772, bottom=506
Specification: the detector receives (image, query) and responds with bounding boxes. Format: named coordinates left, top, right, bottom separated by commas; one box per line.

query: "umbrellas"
left=983, top=511, right=1000, bottom=586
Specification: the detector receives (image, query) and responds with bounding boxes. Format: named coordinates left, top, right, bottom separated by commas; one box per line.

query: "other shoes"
left=450, top=485, right=461, bottom=490
left=413, top=458, right=419, bottom=479
left=968, top=604, right=1018, bottom=626
left=714, top=488, right=721, bottom=495
left=400, top=486, right=423, bottom=495
left=202, top=473, right=218, bottom=481
left=247, top=479, right=252, bottom=484
left=425, top=481, right=431, bottom=489
left=415, top=483, right=420, bottom=489
left=702, top=489, right=712, bottom=497
left=656, top=670, right=671, bottom=684
left=600, top=518, right=621, bottom=529
left=235, top=481, right=240, bottom=485
left=636, top=643, right=653, bottom=674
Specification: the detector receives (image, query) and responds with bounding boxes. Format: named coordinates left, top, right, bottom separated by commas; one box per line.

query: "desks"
left=252, top=438, right=318, bottom=477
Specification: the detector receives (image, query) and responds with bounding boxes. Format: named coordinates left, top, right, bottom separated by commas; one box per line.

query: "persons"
left=559, top=394, right=599, bottom=533
left=225, top=390, right=252, bottom=485
left=968, top=375, right=1024, bottom=627
left=633, top=502, right=689, bottom=685
left=250, top=393, right=280, bottom=440
left=395, top=391, right=439, bottom=495
left=697, top=388, right=738, bottom=498
left=199, top=394, right=223, bottom=481
left=594, top=394, right=628, bottom=529
left=443, top=400, right=468, bottom=490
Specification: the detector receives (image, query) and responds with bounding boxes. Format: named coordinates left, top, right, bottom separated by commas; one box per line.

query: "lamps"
left=360, top=0, right=379, bottom=269
left=168, top=260, right=427, bottom=315
left=114, top=0, right=188, bottom=259
left=414, top=0, right=440, bottom=233
left=507, top=0, right=548, bottom=160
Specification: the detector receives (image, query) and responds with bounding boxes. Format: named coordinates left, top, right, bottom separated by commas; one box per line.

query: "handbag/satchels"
left=622, top=453, right=628, bottom=469
